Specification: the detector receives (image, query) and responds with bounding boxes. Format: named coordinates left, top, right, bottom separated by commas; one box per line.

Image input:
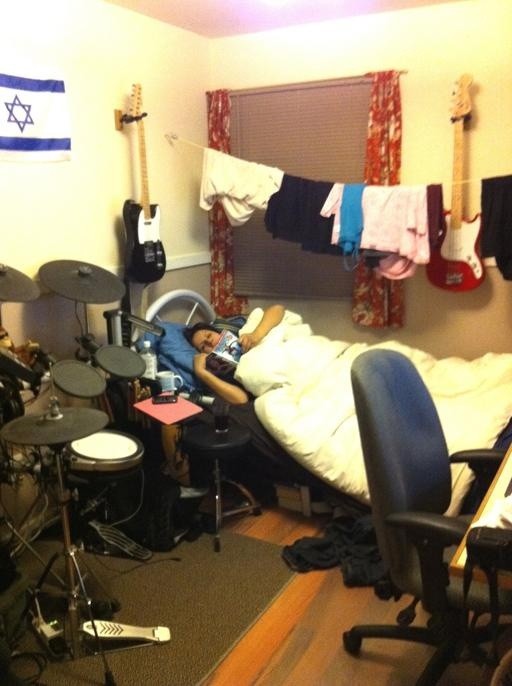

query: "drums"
left=64, top=430, right=146, bottom=471
left=52, top=359, right=107, bottom=399
left=95, top=344, right=146, bottom=379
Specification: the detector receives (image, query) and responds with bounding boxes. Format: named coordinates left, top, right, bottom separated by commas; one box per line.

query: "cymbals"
left=39, top=260, right=126, bottom=303
left=1, top=407, right=109, bottom=446
left=0, top=347, right=41, bottom=385
left=0, top=264, right=41, bottom=301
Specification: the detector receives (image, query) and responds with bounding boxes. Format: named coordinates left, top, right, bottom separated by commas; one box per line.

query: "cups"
left=156, top=371, right=184, bottom=393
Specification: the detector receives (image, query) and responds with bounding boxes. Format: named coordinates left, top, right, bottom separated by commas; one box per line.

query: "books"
left=204, top=329, right=242, bottom=384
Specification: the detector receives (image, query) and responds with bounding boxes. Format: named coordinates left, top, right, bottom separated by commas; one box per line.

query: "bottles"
left=138, top=340, right=158, bottom=380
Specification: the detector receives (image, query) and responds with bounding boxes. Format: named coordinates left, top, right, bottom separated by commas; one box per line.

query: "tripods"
left=5, top=448, right=122, bottom=686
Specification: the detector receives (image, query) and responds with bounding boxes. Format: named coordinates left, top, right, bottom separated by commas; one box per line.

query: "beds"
left=131, top=288, right=512, bottom=519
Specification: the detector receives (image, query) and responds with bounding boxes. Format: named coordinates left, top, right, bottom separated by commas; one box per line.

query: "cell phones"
left=151, top=395, right=178, bottom=404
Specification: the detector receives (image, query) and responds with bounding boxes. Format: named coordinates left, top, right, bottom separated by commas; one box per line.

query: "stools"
left=182, top=424, right=261, bottom=550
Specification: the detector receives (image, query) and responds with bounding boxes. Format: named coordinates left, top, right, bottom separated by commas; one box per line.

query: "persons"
left=181, top=303, right=285, bottom=407
left=209, top=359, right=228, bottom=371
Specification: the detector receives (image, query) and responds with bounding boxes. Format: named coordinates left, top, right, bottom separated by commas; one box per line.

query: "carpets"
left=1, top=528, right=297, bottom=686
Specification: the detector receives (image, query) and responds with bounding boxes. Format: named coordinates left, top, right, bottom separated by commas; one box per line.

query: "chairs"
left=343, top=347, right=512, bottom=685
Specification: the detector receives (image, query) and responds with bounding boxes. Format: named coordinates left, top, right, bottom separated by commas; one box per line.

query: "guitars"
left=426, top=74, right=484, bottom=292
left=123, top=84, right=166, bottom=283
